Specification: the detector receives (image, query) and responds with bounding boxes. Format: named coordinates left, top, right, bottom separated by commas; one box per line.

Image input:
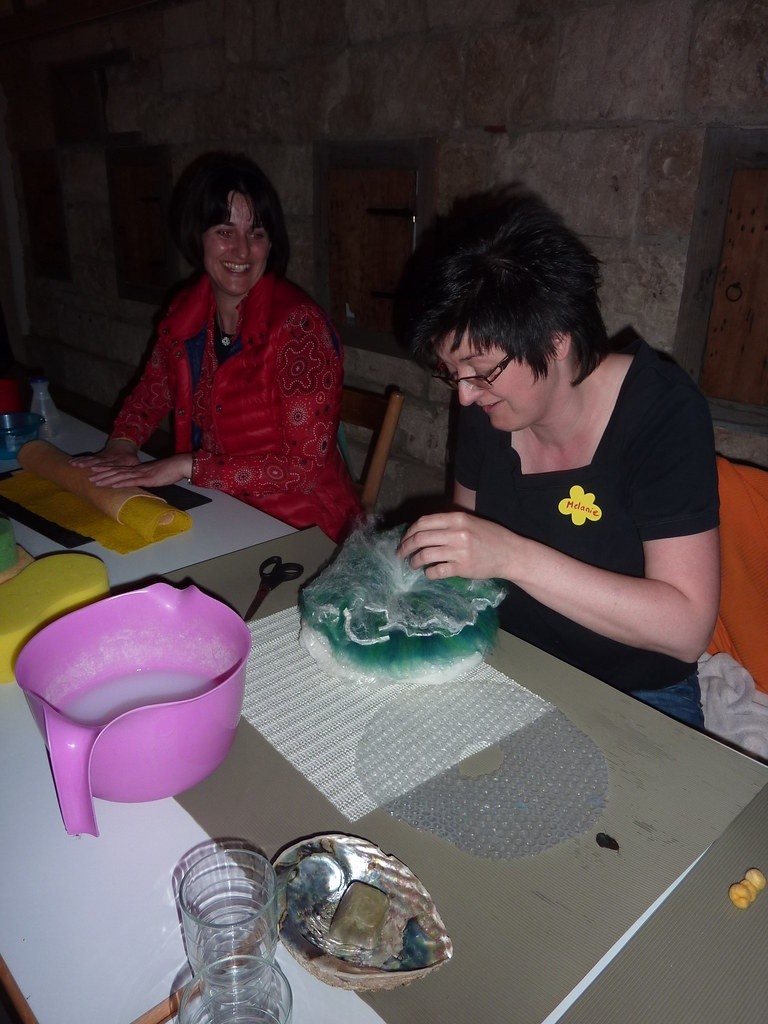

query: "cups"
left=181, top=953, right=293, bottom=1024
left=178, top=848, right=279, bottom=998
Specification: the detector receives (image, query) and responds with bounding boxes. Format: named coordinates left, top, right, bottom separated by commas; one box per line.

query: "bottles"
left=29, top=377, right=62, bottom=449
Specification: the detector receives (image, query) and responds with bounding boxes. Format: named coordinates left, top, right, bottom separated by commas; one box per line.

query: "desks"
left=0, top=408, right=768, bottom=1024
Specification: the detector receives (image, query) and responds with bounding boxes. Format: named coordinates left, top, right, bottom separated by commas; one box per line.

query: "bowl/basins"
left=1, top=414, right=46, bottom=460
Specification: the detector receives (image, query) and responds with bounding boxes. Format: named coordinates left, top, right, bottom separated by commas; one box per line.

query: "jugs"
left=13, top=583, right=250, bottom=836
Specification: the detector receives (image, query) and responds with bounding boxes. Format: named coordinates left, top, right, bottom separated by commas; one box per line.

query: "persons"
left=66, top=150, right=370, bottom=552
left=396, top=184, right=720, bottom=732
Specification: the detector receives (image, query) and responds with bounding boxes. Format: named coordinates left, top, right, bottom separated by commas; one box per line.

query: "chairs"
left=337, top=384, right=404, bottom=516
